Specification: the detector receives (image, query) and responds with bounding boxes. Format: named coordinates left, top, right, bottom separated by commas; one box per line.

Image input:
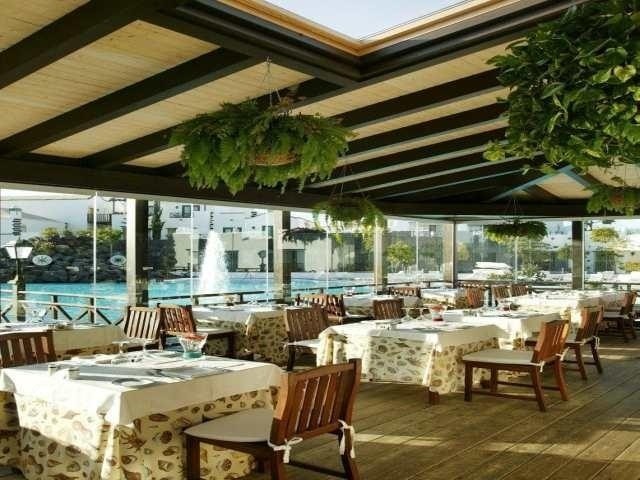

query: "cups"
left=47, top=364, right=57, bottom=376
left=66, top=366, right=80, bottom=381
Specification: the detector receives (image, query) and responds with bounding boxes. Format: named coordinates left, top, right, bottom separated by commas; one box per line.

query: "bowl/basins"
left=441, top=311, right=463, bottom=322
left=429, top=304, right=447, bottom=320
left=176, top=331, right=209, bottom=358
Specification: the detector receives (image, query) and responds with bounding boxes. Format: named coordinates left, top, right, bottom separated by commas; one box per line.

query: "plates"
left=112, top=377, right=154, bottom=388
left=415, top=326, right=440, bottom=333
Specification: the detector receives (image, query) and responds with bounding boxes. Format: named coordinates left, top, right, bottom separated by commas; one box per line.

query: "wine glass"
left=111, top=338, right=156, bottom=369
left=400, top=308, right=428, bottom=324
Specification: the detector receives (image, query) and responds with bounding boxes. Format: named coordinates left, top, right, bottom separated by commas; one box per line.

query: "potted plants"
left=167, top=95, right=391, bottom=255
left=582, top=177, right=640, bottom=218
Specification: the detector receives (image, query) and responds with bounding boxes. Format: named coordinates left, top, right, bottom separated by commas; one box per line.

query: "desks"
left=2, top=350, right=284, bottom=480
left=317, top=318, right=511, bottom=404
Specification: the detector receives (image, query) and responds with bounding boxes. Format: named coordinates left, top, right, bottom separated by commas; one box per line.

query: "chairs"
left=461, top=318, right=569, bottom=411
left=1, top=280, right=639, bottom=379
left=184, top=358, right=362, bottom=480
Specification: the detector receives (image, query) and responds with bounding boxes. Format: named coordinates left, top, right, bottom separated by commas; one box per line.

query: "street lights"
left=2, top=202, right=34, bottom=324
